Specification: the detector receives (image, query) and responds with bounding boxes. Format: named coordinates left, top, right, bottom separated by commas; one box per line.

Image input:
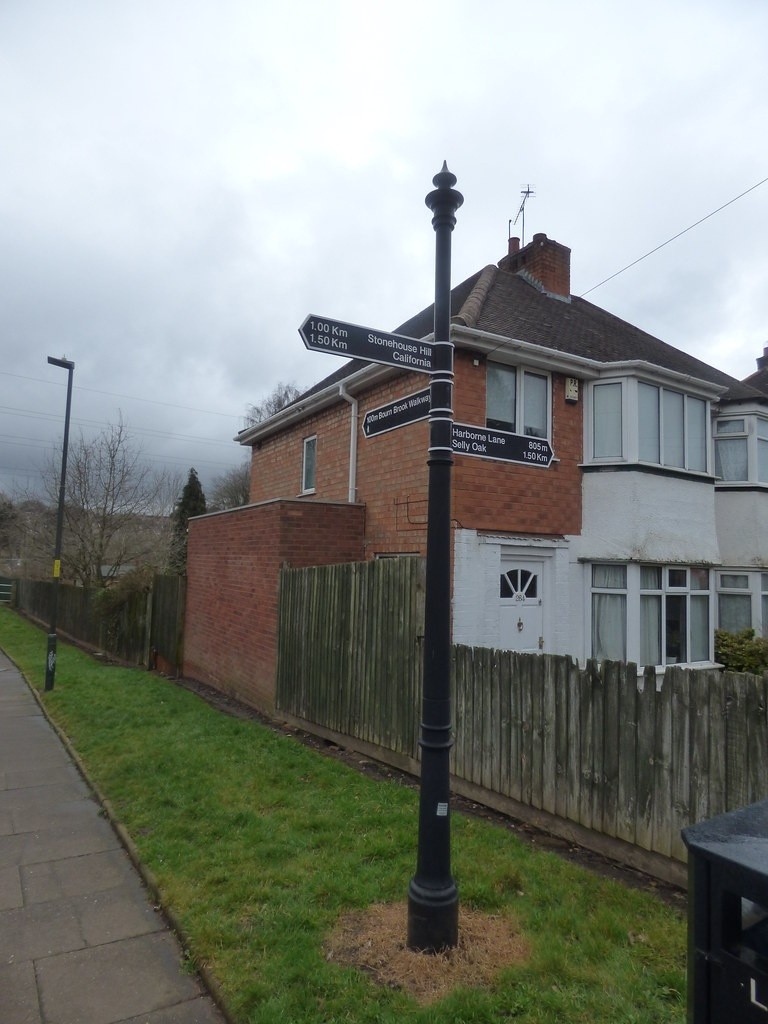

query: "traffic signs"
left=362, top=386, right=431, bottom=440
left=451, top=422, right=555, bottom=468
left=298, top=314, right=433, bottom=375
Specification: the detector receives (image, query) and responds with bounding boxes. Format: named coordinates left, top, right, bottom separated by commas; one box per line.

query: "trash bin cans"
left=679, top=801, right=768, bottom=1023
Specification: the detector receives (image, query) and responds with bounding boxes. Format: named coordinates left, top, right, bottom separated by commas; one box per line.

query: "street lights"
left=43, top=355, right=76, bottom=692
